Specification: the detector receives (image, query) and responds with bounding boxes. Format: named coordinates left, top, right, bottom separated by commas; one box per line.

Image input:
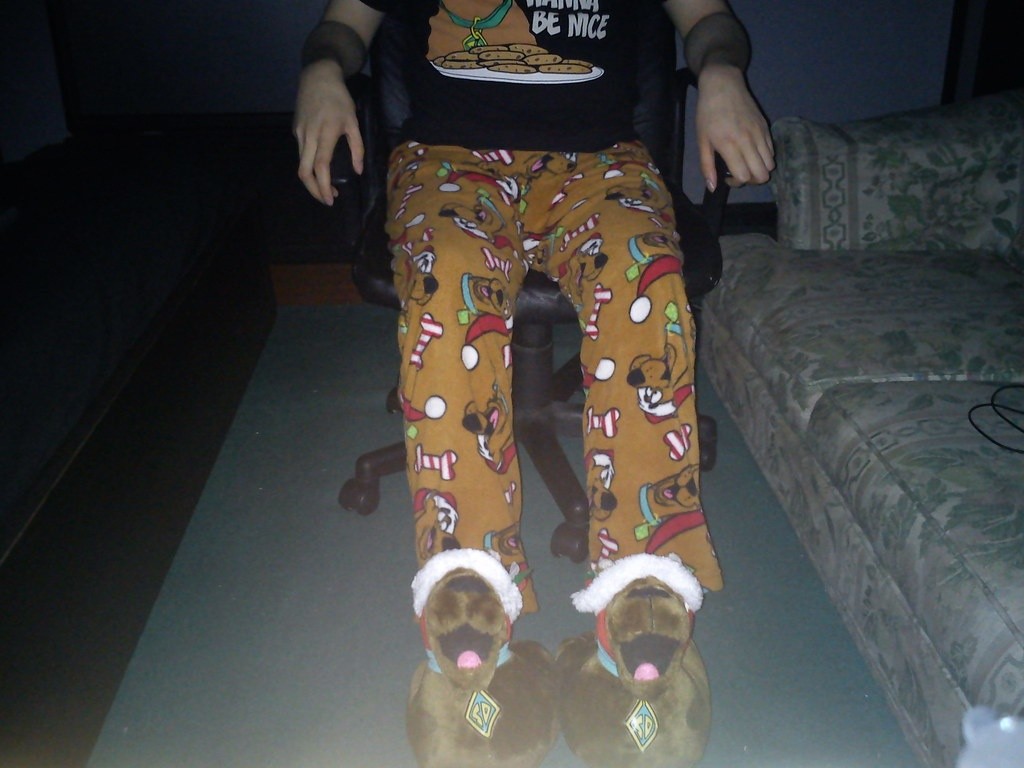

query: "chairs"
left=340, top=62, right=732, bottom=563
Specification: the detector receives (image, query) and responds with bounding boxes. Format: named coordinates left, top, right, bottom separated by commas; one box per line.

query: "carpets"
left=91, top=302, right=926, bottom=767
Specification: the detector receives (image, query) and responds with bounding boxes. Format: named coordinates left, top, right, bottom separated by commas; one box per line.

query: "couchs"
left=689, top=93, right=1024, bottom=768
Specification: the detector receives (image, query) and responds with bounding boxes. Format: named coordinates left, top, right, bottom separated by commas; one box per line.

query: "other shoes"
left=406, top=546, right=556, bottom=768
left=556, top=555, right=709, bottom=768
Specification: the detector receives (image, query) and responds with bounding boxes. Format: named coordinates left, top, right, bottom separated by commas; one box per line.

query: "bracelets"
left=683, top=13, right=751, bottom=73
left=301, top=21, right=366, bottom=78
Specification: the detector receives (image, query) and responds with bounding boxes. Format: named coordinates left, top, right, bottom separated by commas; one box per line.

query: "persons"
left=292, top=0, right=775, bottom=768
